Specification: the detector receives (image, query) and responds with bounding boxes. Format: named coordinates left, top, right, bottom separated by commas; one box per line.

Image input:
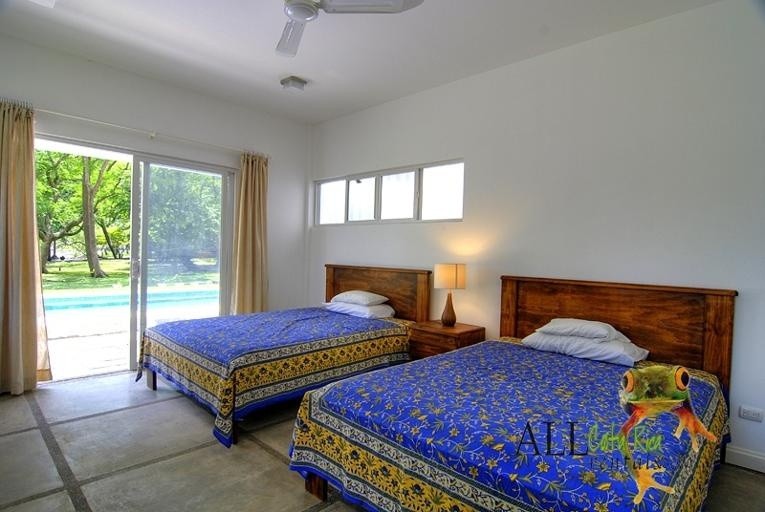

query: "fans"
left=274, top=0, right=422, bottom=58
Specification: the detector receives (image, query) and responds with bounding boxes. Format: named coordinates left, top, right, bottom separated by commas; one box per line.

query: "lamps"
left=432, top=264, right=467, bottom=326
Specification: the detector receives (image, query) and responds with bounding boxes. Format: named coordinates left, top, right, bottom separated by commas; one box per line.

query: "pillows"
left=520, top=315, right=650, bottom=367
left=323, top=289, right=395, bottom=321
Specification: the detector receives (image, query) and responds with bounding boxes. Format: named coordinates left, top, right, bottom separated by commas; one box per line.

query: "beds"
left=139, top=266, right=432, bottom=445
left=296, top=277, right=739, bottom=512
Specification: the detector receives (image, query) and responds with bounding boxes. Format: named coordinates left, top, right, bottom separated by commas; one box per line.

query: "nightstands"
left=409, top=319, right=485, bottom=360
